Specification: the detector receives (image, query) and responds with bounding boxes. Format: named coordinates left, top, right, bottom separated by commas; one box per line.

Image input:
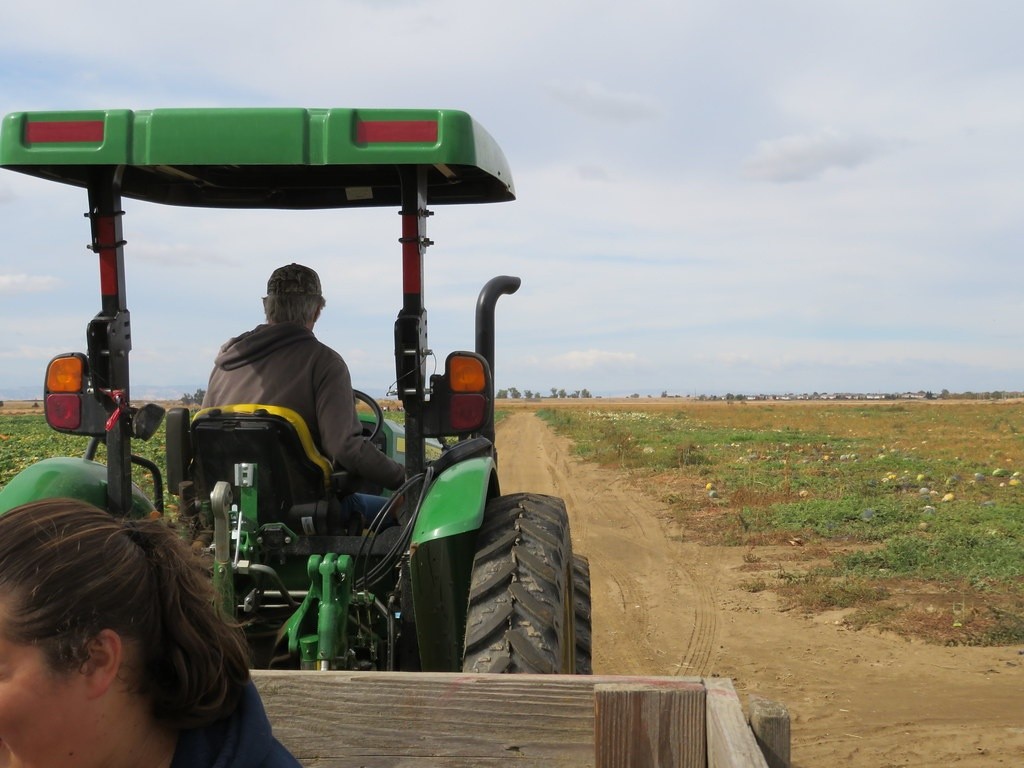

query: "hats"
left=267, top=263, right=322, bottom=296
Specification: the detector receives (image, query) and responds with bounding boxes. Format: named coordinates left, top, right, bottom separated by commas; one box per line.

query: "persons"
left=0, top=496, right=300, bottom=768
left=197, top=262, right=405, bottom=534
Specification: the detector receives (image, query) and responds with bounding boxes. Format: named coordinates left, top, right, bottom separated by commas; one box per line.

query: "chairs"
left=188, top=404, right=333, bottom=536
